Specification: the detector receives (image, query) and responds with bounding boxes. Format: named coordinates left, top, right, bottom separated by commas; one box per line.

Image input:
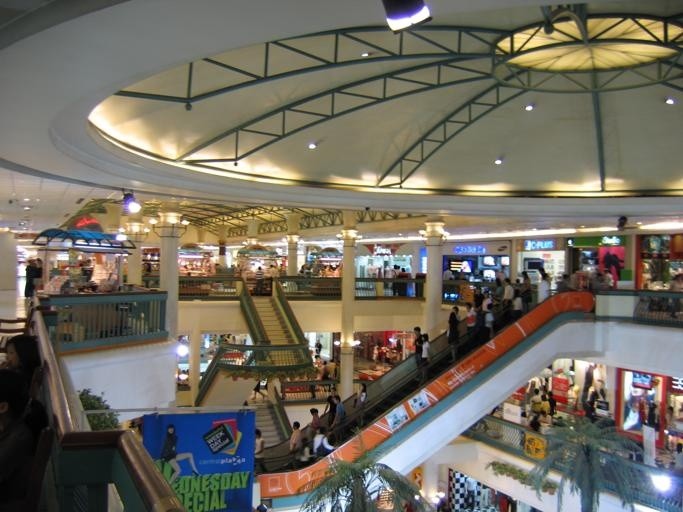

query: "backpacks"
left=476, top=311, right=491, bottom=327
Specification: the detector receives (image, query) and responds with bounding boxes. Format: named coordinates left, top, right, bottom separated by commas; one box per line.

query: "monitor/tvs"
left=479, top=255, right=510, bottom=283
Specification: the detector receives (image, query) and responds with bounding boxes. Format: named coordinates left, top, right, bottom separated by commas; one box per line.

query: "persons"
left=669, top=274, right=682, bottom=318
left=404, top=478, right=512, bottom=512
left=254, top=429, right=268, bottom=473
left=623, top=395, right=657, bottom=434
left=602, top=267, right=614, bottom=289
left=212, top=334, right=248, bottom=345
left=230, top=259, right=339, bottom=296
left=144, top=262, right=151, bottom=288
left=0, top=334, right=41, bottom=503
left=288, top=337, right=347, bottom=469
left=160, top=424, right=199, bottom=486
left=356, top=382, right=368, bottom=427
left=420, top=333, right=434, bottom=384
left=24, top=258, right=43, bottom=300
left=581, top=379, right=609, bottom=411
left=251, top=381, right=265, bottom=400
left=413, top=326, right=423, bottom=379
left=520, top=377, right=557, bottom=431
left=378, top=264, right=578, bottom=363
left=372, top=342, right=382, bottom=371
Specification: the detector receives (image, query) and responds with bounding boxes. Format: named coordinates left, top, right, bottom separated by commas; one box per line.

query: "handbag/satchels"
left=317, top=444, right=334, bottom=456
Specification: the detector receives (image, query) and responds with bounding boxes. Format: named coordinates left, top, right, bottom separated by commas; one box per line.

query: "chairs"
left=0, top=316, right=55, bottom=512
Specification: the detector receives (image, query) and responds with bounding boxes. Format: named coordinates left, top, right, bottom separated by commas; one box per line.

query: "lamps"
left=380, top=1, right=433, bottom=35
